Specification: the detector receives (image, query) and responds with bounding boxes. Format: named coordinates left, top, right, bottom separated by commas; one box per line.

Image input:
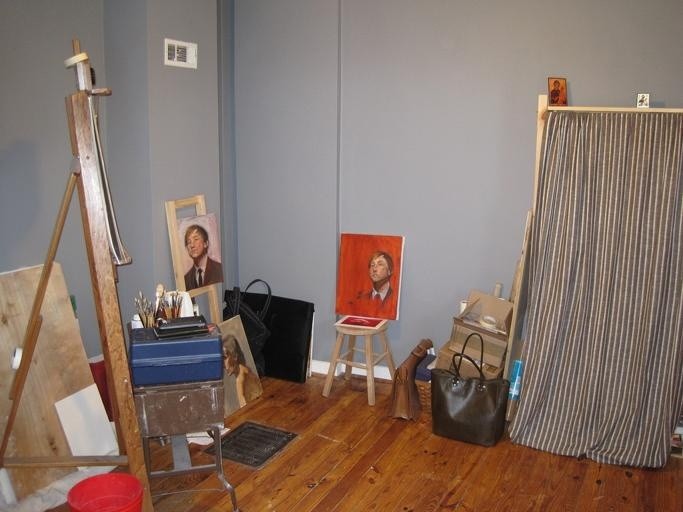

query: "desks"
left=132, top=377, right=239, bottom=512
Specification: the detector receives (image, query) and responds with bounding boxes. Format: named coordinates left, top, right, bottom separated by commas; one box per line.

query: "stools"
left=321, top=314, right=397, bottom=407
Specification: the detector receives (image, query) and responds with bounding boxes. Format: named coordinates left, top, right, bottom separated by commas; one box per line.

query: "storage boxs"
left=435, top=342, right=504, bottom=380
left=446, top=288, right=513, bottom=368
left=128, top=323, right=223, bottom=386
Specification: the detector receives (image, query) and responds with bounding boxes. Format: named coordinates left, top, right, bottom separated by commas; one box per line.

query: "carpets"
left=199, top=419, right=298, bottom=472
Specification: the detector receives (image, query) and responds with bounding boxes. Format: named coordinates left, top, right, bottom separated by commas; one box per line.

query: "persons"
left=550, top=80, right=561, bottom=103
left=221, top=334, right=263, bottom=409
left=352, top=250, right=398, bottom=319
left=184, top=225, right=223, bottom=292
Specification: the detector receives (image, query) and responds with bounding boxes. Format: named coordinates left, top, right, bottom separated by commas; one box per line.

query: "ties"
left=198, top=268, right=203, bottom=286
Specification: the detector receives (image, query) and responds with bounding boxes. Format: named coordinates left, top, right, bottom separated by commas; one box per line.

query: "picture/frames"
left=546, top=76, right=569, bottom=108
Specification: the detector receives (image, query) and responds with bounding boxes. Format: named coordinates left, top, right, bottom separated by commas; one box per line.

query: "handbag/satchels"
left=225, top=281, right=273, bottom=378
left=431, top=332, right=510, bottom=446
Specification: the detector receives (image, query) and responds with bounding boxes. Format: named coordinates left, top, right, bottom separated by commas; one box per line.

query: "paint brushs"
left=124, top=291, right=157, bottom=328
left=156, top=291, right=183, bottom=319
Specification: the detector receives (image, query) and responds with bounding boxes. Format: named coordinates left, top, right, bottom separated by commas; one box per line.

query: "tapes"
left=478, top=314, right=498, bottom=329
